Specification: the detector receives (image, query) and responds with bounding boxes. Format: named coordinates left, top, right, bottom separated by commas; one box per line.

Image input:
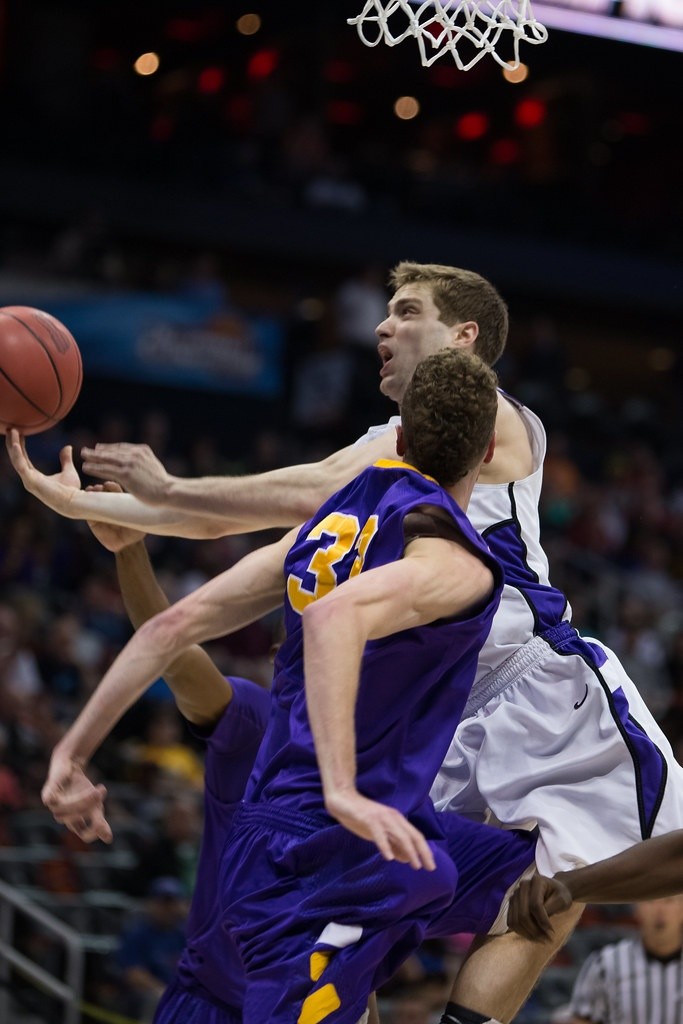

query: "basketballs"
left=1, top=305, right=88, bottom=440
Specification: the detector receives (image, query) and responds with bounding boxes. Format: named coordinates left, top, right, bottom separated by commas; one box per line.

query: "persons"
left=568, top=894, right=682, bottom=1024
left=38, top=348, right=514, bottom=1024
left=506, top=826, right=682, bottom=947
left=0, top=260, right=683, bottom=1024
left=77, top=480, right=538, bottom=1023
